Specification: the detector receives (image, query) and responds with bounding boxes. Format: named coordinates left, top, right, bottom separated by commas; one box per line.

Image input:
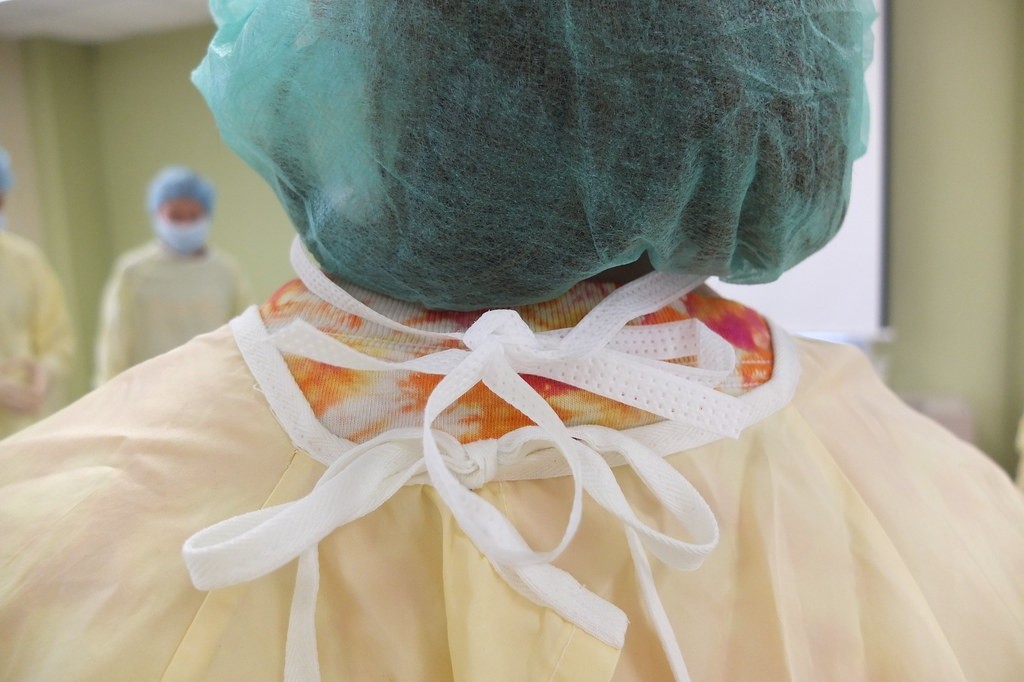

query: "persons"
left=0, top=148, right=73, bottom=443
left=0, top=0, right=1024, bottom=681
left=95, top=166, right=242, bottom=393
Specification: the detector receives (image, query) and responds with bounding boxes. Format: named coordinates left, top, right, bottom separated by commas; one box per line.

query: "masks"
left=151, top=211, right=210, bottom=255
